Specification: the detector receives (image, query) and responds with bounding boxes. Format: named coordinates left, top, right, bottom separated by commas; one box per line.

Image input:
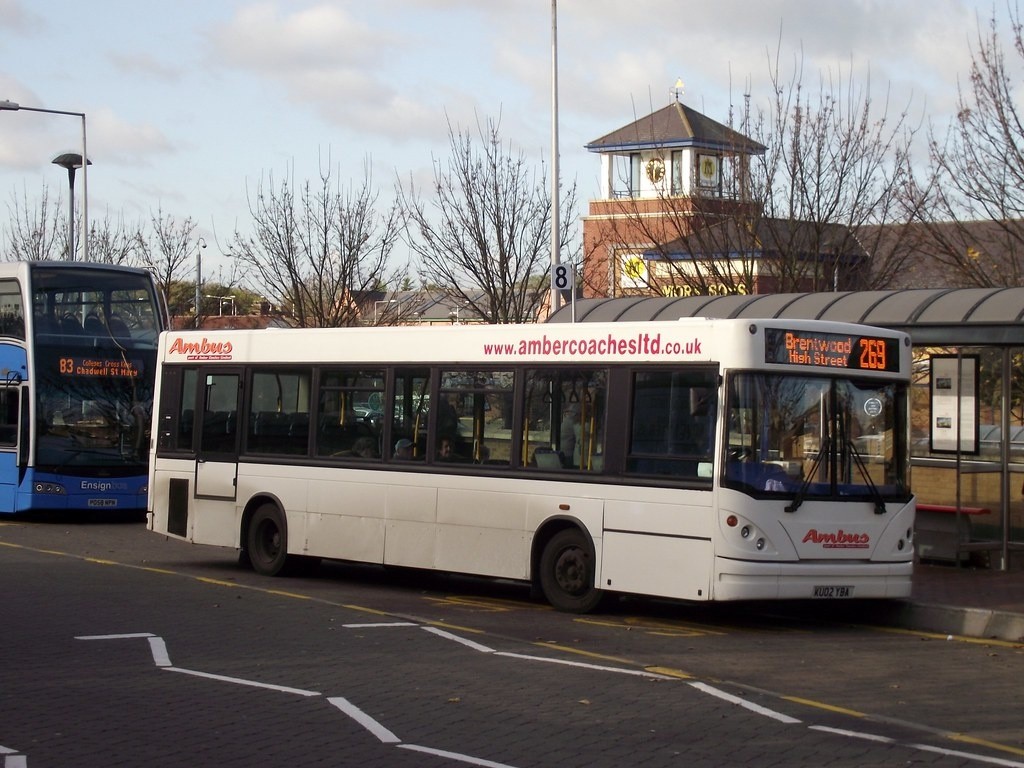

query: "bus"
left=145, top=316, right=917, bottom=615
left=0, top=261, right=164, bottom=523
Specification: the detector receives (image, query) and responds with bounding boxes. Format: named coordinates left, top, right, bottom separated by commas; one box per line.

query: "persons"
left=350, top=435, right=459, bottom=464
left=560, top=401, right=598, bottom=470
left=423, top=392, right=458, bottom=438
left=126, top=405, right=151, bottom=446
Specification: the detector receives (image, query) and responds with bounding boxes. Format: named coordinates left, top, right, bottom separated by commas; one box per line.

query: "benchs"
left=914, top=503, right=992, bottom=543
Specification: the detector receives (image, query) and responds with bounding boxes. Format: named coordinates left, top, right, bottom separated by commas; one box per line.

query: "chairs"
left=182, top=408, right=403, bottom=456
left=534, top=449, right=565, bottom=469
left=39, top=313, right=132, bottom=337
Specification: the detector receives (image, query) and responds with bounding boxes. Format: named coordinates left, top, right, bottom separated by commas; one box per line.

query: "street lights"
left=220, top=296, right=224, bottom=317
left=52, top=154, right=92, bottom=262
left=0, top=100, right=87, bottom=261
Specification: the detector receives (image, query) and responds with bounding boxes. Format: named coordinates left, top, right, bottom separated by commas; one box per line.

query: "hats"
left=395, top=439, right=417, bottom=451
